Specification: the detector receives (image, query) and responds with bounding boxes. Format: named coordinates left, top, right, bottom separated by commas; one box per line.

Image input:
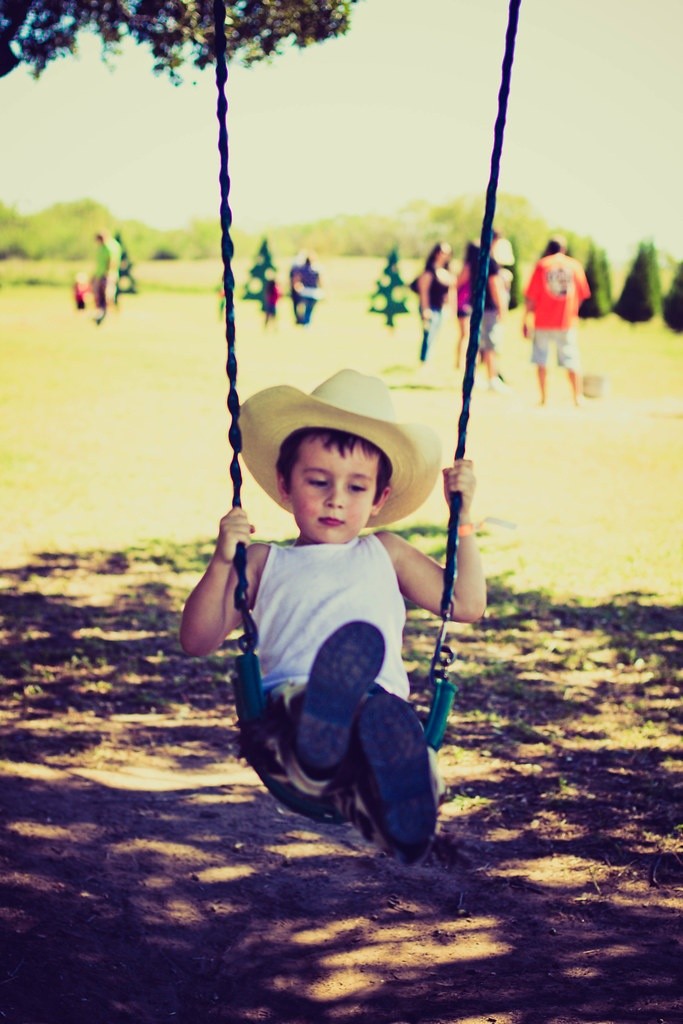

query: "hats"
left=237, top=368, right=441, bottom=528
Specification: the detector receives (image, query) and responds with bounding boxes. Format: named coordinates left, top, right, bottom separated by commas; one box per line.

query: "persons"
left=179, top=368, right=488, bottom=865
left=260, top=254, right=324, bottom=327
left=73, top=235, right=122, bottom=324
left=416, top=228, right=590, bottom=405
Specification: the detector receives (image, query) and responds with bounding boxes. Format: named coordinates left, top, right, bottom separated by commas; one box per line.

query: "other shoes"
left=294, top=620, right=386, bottom=767
left=359, top=694, right=437, bottom=856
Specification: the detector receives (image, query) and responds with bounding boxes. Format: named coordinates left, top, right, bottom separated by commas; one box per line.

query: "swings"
left=212, top=1, right=522, bottom=823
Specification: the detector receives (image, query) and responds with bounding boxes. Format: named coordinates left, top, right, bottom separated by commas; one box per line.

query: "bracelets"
left=457, top=524, right=475, bottom=537
